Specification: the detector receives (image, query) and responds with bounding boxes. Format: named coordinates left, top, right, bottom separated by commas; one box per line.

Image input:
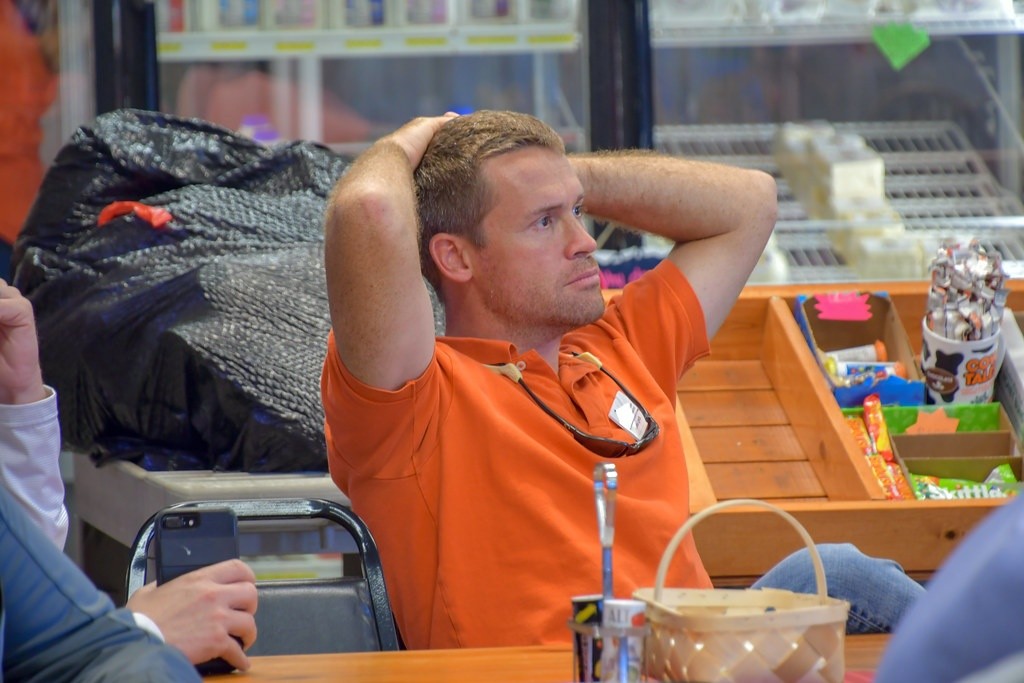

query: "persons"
left=0, top=277, right=259, bottom=683
left=323, top=113, right=924, bottom=651
left=873, top=489, right=1023, bottom=683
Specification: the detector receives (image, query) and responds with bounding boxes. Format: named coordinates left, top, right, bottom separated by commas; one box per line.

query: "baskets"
left=632, top=499, right=850, bottom=683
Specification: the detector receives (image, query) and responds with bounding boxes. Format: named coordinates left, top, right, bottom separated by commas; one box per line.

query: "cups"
left=920, top=316, right=1006, bottom=406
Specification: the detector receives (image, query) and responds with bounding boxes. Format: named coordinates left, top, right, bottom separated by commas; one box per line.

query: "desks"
left=202, top=634, right=893, bottom=683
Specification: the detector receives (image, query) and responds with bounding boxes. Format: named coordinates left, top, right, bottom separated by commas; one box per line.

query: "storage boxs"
left=840, top=402, right=1024, bottom=499
left=792, top=289, right=923, bottom=408
left=674, top=282, right=1024, bottom=578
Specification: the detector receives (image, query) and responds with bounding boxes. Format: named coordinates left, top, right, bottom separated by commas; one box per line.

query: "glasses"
left=496, top=352, right=660, bottom=458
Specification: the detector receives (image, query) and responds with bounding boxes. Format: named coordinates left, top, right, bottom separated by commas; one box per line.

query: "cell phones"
left=154, top=507, right=243, bottom=676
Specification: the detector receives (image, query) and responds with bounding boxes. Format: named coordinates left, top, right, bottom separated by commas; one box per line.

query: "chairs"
left=117, top=495, right=399, bottom=655
